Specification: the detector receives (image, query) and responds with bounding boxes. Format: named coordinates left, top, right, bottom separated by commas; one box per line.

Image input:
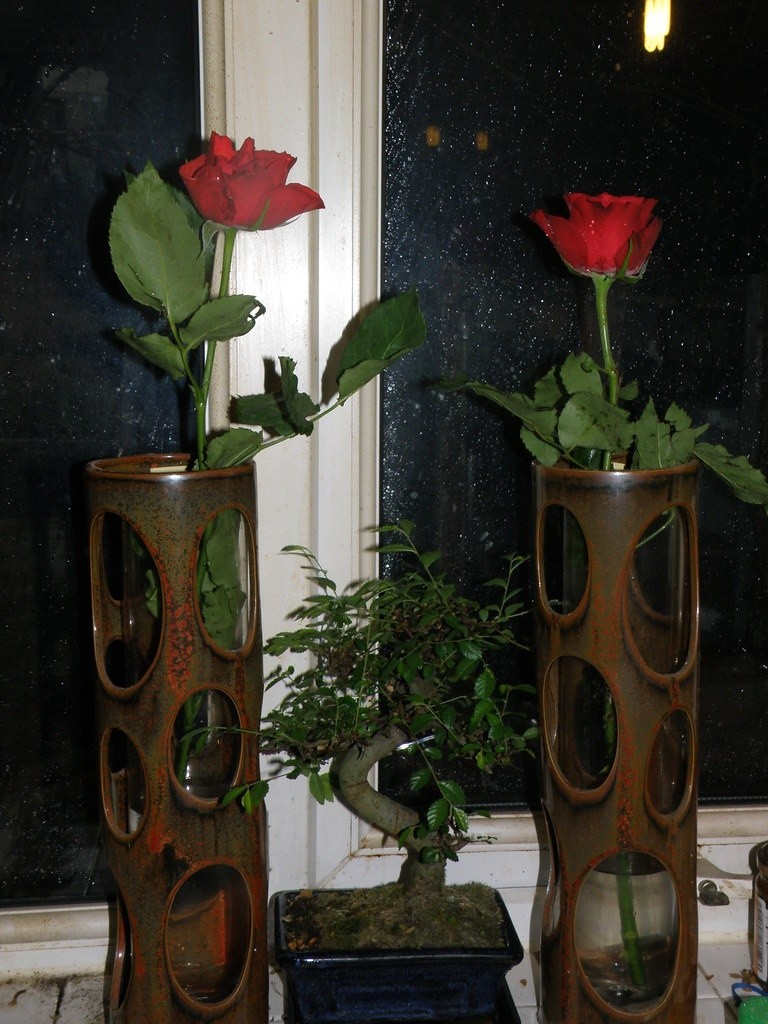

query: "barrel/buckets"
left=751, top=840, right=768, bottom=985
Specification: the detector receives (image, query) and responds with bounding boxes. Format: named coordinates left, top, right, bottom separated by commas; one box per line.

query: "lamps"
left=642, top=0, right=672, bottom=53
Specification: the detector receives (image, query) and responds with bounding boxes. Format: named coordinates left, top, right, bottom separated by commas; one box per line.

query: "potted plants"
left=269, top=511, right=530, bottom=1024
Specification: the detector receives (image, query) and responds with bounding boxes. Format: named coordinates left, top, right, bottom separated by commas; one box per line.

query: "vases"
left=524, top=450, right=700, bottom=1024
left=86, top=452, right=269, bottom=1024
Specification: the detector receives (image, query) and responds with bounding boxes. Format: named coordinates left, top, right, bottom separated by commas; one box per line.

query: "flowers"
left=460, top=194, right=768, bottom=516
left=100, top=126, right=428, bottom=468
